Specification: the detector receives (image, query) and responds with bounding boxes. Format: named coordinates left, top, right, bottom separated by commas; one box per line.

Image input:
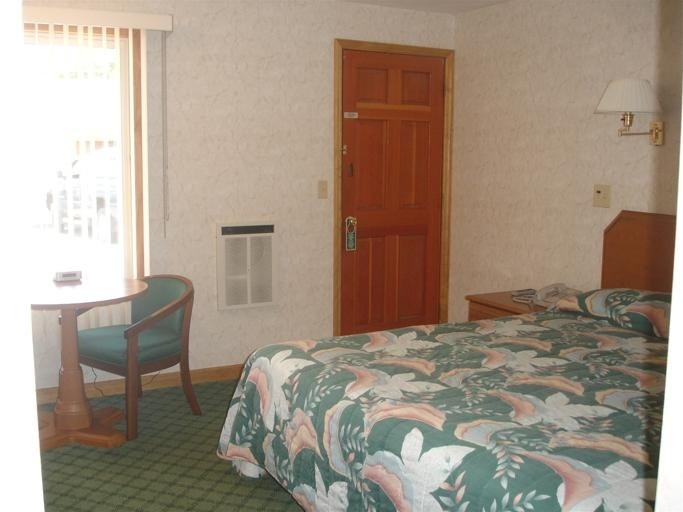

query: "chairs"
left=58, top=274, right=203, bottom=441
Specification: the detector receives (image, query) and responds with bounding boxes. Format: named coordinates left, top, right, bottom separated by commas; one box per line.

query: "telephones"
left=512, top=283, right=584, bottom=309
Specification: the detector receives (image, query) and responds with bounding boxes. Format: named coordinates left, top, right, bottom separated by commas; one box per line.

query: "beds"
left=214, top=210, right=675, bottom=512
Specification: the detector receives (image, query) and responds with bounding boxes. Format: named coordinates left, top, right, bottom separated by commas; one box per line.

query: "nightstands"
left=465, top=288, right=554, bottom=320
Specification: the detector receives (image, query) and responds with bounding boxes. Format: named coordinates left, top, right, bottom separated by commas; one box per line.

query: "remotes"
left=511, top=289, right=535, bottom=295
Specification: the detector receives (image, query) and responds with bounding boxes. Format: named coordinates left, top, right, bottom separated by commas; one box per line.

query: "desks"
left=31, top=274, right=149, bottom=449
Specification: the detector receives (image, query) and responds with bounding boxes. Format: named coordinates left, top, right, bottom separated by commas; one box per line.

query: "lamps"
left=592, top=77, right=665, bottom=148
